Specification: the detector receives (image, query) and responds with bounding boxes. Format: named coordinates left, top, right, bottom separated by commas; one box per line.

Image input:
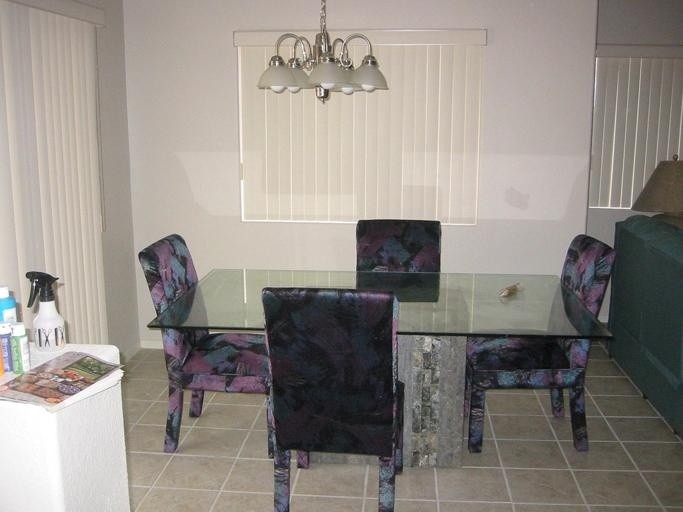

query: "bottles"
left=0, top=286, right=32, bottom=375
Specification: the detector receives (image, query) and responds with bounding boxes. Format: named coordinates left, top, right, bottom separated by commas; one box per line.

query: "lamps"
left=630, top=154, right=682, bottom=215
left=256, top=3, right=389, bottom=105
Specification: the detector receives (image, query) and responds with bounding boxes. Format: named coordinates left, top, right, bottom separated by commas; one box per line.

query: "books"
left=0, top=350, right=125, bottom=414
